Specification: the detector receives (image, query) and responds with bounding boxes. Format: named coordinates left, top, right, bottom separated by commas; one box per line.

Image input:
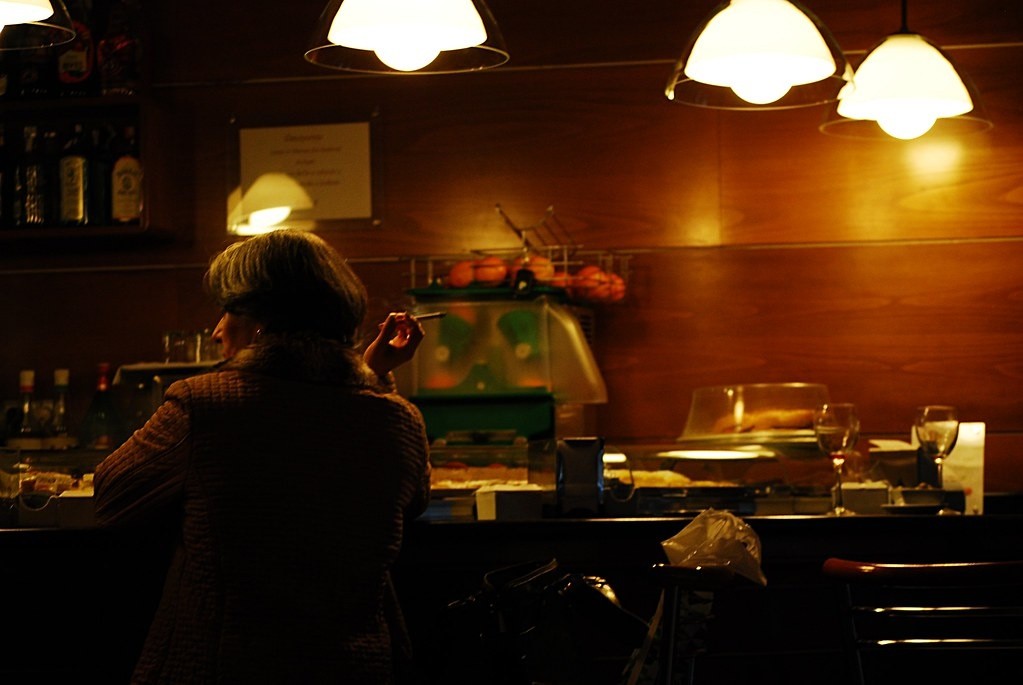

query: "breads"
left=710, top=409, right=833, bottom=433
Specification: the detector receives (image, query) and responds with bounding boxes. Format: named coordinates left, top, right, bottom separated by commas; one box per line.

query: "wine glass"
left=813, top=403, right=860, bottom=517
left=915, top=405, right=960, bottom=517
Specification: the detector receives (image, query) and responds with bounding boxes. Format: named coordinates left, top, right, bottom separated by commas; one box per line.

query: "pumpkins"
left=443, top=254, right=624, bottom=303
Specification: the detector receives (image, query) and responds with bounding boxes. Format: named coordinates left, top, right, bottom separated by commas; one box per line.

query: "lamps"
left=304, top=0, right=510, bottom=74
left=818, top=0, right=993, bottom=148
left=665, top=0, right=856, bottom=112
left=0, top=0, right=79, bottom=55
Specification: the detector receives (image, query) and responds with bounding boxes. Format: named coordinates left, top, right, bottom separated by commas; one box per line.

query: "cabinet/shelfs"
left=0, top=90, right=197, bottom=246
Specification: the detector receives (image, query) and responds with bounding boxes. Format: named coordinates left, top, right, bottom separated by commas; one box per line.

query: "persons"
left=93, top=229, right=432, bottom=685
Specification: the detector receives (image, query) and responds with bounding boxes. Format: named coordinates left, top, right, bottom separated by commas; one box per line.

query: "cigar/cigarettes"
left=414, top=312, right=447, bottom=321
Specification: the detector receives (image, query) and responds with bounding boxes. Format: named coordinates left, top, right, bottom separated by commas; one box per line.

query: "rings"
left=407, top=335, right=410, bottom=339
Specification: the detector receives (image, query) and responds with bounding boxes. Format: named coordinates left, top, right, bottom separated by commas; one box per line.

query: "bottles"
left=103, top=126, right=148, bottom=226
left=50, top=369, right=76, bottom=439
left=58, top=124, right=91, bottom=227
left=14, top=370, right=41, bottom=439
left=12, top=126, right=46, bottom=229
left=84, top=363, right=116, bottom=450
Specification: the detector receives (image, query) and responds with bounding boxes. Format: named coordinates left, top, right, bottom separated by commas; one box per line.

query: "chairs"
left=819, top=557, right=1023, bottom=685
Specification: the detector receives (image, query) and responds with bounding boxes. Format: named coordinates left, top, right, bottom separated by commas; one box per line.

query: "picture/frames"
left=226, top=106, right=386, bottom=233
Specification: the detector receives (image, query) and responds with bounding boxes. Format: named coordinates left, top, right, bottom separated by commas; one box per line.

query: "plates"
left=880, top=504, right=946, bottom=515
